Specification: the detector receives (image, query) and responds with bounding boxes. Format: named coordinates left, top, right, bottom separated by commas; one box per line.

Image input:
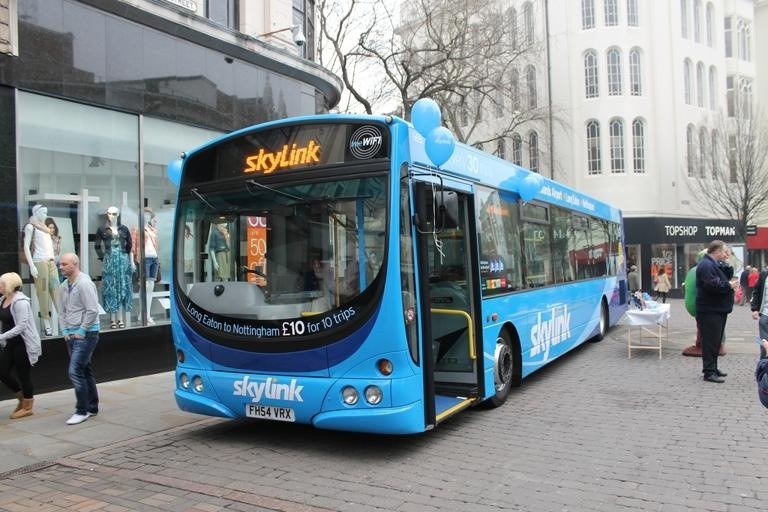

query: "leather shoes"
left=704, top=368, right=728, bottom=384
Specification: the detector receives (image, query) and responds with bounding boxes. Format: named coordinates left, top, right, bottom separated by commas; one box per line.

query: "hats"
left=138, top=207, right=156, bottom=217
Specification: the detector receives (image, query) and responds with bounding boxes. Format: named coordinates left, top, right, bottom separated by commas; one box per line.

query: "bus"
left=161, top=114, right=633, bottom=440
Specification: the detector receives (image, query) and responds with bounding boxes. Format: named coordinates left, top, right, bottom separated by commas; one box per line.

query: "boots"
left=9, top=390, right=34, bottom=419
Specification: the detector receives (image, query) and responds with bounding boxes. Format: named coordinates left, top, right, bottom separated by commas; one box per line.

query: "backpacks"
left=756, top=357, right=768, bottom=408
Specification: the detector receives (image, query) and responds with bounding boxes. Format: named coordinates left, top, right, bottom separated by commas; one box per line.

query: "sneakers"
left=44, top=326, right=52, bottom=337
left=65, top=411, right=98, bottom=425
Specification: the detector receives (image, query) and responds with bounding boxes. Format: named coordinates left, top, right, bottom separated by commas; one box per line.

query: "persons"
left=654, top=267, right=671, bottom=303
left=94, top=207, right=132, bottom=328
left=696, top=240, right=738, bottom=382
left=24, top=205, right=60, bottom=336
left=45, top=218, right=60, bottom=256
left=628, top=265, right=640, bottom=304
left=740, top=265, right=768, bottom=409
left=209, top=222, right=230, bottom=282
left=131, top=207, right=158, bottom=326
left=184, top=225, right=194, bottom=272
left=57, top=253, right=99, bottom=424
left=0, top=272, right=42, bottom=419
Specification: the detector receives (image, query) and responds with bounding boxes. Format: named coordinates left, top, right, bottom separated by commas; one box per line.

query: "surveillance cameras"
left=295, top=32, right=306, bottom=46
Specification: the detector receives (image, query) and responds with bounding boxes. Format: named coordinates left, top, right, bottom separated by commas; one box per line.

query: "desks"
left=617, top=301, right=671, bottom=360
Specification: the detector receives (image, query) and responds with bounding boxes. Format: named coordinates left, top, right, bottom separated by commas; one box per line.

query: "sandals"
left=110, top=319, right=124, bottom=329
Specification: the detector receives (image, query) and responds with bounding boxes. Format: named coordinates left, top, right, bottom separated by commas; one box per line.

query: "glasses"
left=107, top=212, right=117, bottom=216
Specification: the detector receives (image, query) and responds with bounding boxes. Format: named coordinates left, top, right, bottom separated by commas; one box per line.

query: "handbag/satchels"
left=19, top=222, right=36, bottom=265
left=154, top=262, right=162, bottom=283
